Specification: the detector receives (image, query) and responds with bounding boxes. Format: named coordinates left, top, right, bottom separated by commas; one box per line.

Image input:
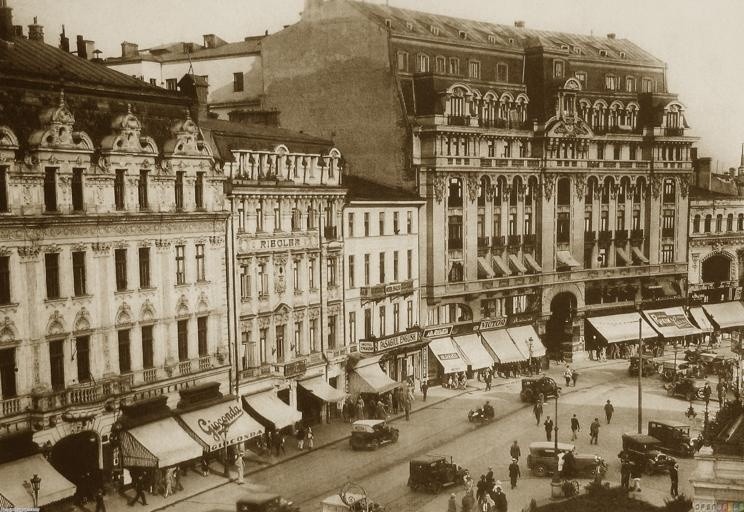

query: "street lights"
left=528, top=337, right=533, bottom=366
left=222, top=418, right=230, bottom=478
left=401, top=367, right=410, bottom=421
left=30, top=474, right=41, bottom=512
left=704, top=382, right=712, bottom=446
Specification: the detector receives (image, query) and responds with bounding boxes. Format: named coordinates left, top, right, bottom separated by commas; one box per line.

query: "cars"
left=348, top=419, right=399, bottom=452
left=619, top=419, right=699, bottom=473
left=406, top=455, right=470, bottom=495
left=237, top=493, right=300, bottom=512
left=627, top=347, right=734, bottom=401
left=319, top=492, right=386, bottom=512
left=527, top=441, right=608, bottom=478
left=521, top=377, right=561, bottom=404
